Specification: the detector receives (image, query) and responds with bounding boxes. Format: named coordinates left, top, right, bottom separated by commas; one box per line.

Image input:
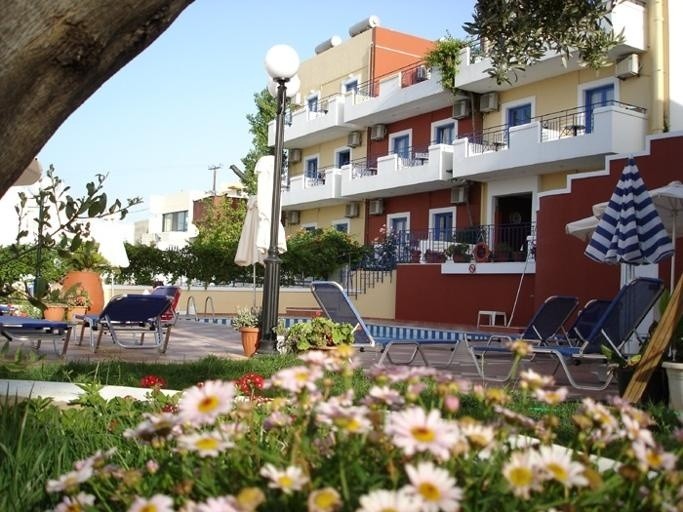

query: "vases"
left=405, top=242, right=526, bottom=265
left=43, top=305, right=68, bottom=321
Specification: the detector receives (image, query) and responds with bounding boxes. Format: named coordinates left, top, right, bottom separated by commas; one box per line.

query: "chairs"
left=140, top=286, right=182, bottom=347
left=472, top=293, right=580, bottom=359
left=470, top=276, right=668, bottom=393
left=310, top=278, right=461, bottom=373
left=547, top=297, right=610, bottom=348
left=72, top=293, right=176, bottom=353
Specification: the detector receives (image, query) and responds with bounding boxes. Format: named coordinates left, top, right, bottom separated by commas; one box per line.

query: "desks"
left=558, top=125, right=586, bottom=137
left=481, top=139, right=507, bottom=152
left=415, top=157, right=429, bottom=167
left=364, top=168, right=377, bottom=175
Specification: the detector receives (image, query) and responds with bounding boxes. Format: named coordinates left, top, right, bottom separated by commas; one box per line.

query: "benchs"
left=0, top=314, right=72, bottom=355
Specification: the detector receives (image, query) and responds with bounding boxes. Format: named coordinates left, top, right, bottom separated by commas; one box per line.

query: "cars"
left=0, top=304, right=18, bottom=316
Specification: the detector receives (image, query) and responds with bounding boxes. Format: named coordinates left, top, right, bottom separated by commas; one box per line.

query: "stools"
left=476, top=309, right=506, bottom=329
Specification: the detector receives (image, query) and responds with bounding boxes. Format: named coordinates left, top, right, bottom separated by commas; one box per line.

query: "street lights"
left=246, top=40, right=303, bottom=362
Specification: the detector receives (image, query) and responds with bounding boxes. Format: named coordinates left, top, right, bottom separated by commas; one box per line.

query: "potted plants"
left=288, top=316, right=362, bottom=360
left=230, top=304, right=267, bottom=357
left=65, top=283, right=93, bottom=321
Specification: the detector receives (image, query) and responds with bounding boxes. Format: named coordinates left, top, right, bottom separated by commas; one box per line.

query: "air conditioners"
left=288, top=149, right=300, bottom=163
left=480, top=92, right=500, bottom=113
left=347, top=132, right=361, bottom=148
left=452, top=99, right=470, bottom=119
left=450, top=188, right=467, bottom=204
left=415, top=65, right=427, bottom=79
left=614, top=53, right=640, bottom=80
left=368, top=200, right=383, bottom=214
left=344, top=202, right=358, bottom=217
left=287, top=211, right=299, bottom=225
left=370, top=125, right=387, bottom=140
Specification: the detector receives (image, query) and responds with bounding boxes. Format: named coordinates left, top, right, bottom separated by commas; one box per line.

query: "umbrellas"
left=565, top=151, right=683, bottom=351
left=254, top=155, right=287, bottom=267
left=97, top=217, right=130, bottom=295
left=233, top=195, right=265, bottom=308
